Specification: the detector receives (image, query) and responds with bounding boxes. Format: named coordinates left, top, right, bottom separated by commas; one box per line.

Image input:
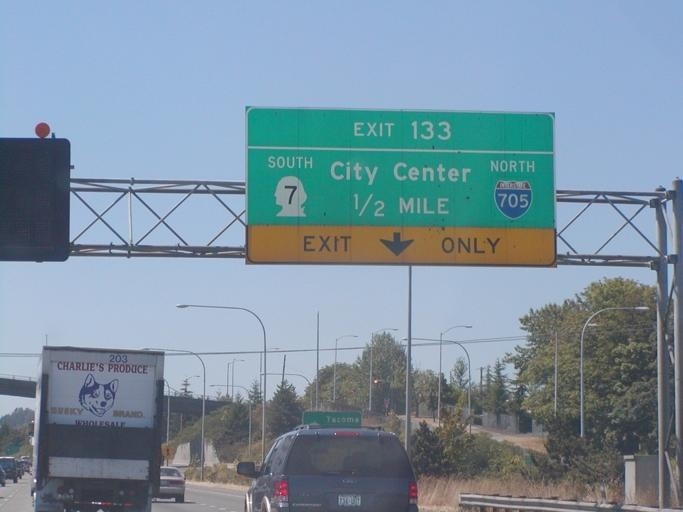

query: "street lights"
left=177, top=304, right=267, bottom=462
left=144, top=347, right=206, bottom=481
left=369, top=327, right=399, bottom=412
left=333, top=333, right=358, bottom=402
left=580, top=305, right=651, bottom=436
left=438, top=324, right=473, bottom=425
left=555, top=322, right=597, bottom=424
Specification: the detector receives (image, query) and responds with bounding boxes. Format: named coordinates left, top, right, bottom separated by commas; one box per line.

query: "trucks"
left=29, top=345, right=165, bottom=511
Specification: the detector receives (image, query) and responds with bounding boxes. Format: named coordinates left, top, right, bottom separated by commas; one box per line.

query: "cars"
left=158, top=467, right=185, bottom=503
left=0, top=455, right=31, bottom=486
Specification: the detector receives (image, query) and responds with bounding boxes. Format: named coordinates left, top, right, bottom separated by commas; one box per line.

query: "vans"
left=237, top=428, right=418, bottom=512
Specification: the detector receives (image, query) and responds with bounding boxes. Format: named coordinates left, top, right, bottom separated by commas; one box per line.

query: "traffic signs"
left=244, top=105, right=557, bottom=265
